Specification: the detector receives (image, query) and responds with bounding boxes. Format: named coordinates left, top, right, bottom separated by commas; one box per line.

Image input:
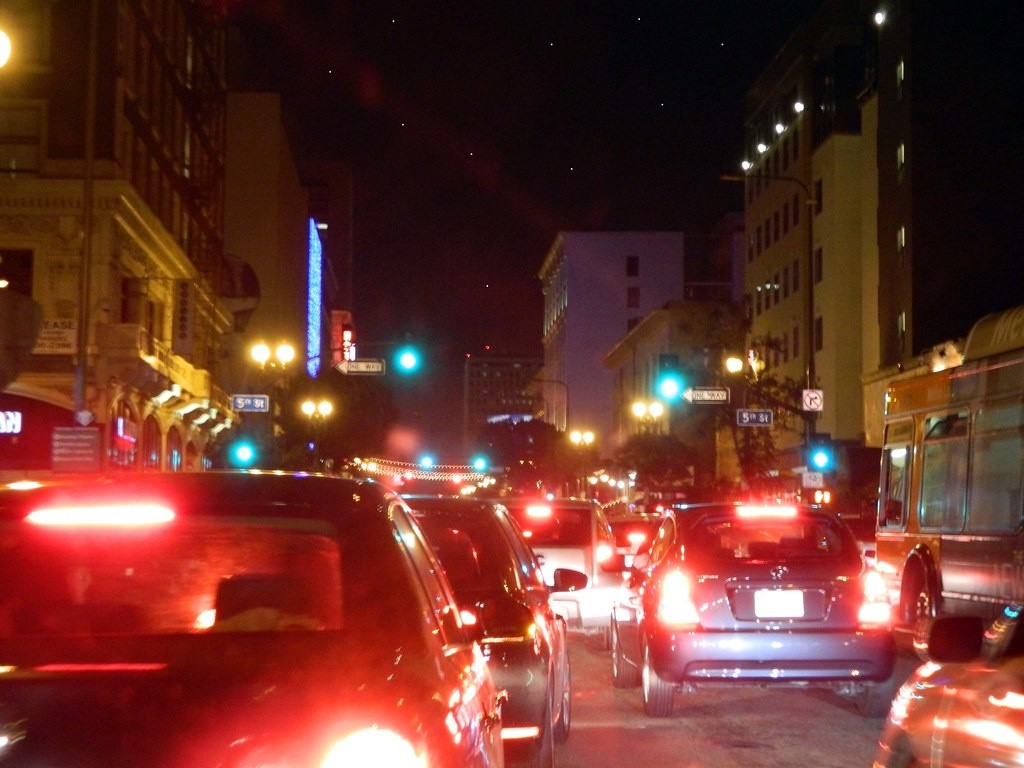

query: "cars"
left=0, top=465, right=539, bottom=768
left=598, top=499, right=903, bottom=720
left=871, top=587, right=1024, bottom=767
left=164, top=469, right=678, bottom=768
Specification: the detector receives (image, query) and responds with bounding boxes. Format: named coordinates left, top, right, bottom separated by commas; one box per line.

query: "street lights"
left=251, top=340, right=296, bottom=470
left=530, top=375, right=572, bottom=436
left=301, top=400, right=334, bottom=472
left=717, top=172, right=817, bottom=504
left=569, top=429, right=595, bottom=498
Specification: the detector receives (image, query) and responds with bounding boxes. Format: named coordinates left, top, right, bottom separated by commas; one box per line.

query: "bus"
left=873, top=346, right=1024, bottom=664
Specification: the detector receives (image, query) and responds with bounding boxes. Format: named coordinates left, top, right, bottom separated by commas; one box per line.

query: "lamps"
left=939, top=343, right=948, bottom=360
left=898, top=362, right=905, bottom=373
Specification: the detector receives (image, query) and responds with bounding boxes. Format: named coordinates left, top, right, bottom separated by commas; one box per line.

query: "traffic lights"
left=681, top=385, right=732, bottom=406
left=396, top=341, right=415, bottom=386
left=473, top=451, right=486, bottom=473
left=810, top=432, right=832, bottom=472
left=237, top=428, right=254, bottom=469
left=659, top=353, right=679, bottom=401
left=421, top=448, right=433, bottom=471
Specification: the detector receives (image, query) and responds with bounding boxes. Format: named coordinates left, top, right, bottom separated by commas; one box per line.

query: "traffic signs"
left=334, top=356, right=386, bottom=376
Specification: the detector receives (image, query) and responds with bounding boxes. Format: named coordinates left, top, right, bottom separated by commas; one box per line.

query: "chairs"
left=697, top=532, right=735, bottom=558
left=439, top=538, right=480, bottom=586
left=558, top=522, right=583, bottom=544
left=779, top=537, right=804, bottom=557
left=747, top=541, right=779, bottom=557
left=213, top=570, right=313, bottom=619
